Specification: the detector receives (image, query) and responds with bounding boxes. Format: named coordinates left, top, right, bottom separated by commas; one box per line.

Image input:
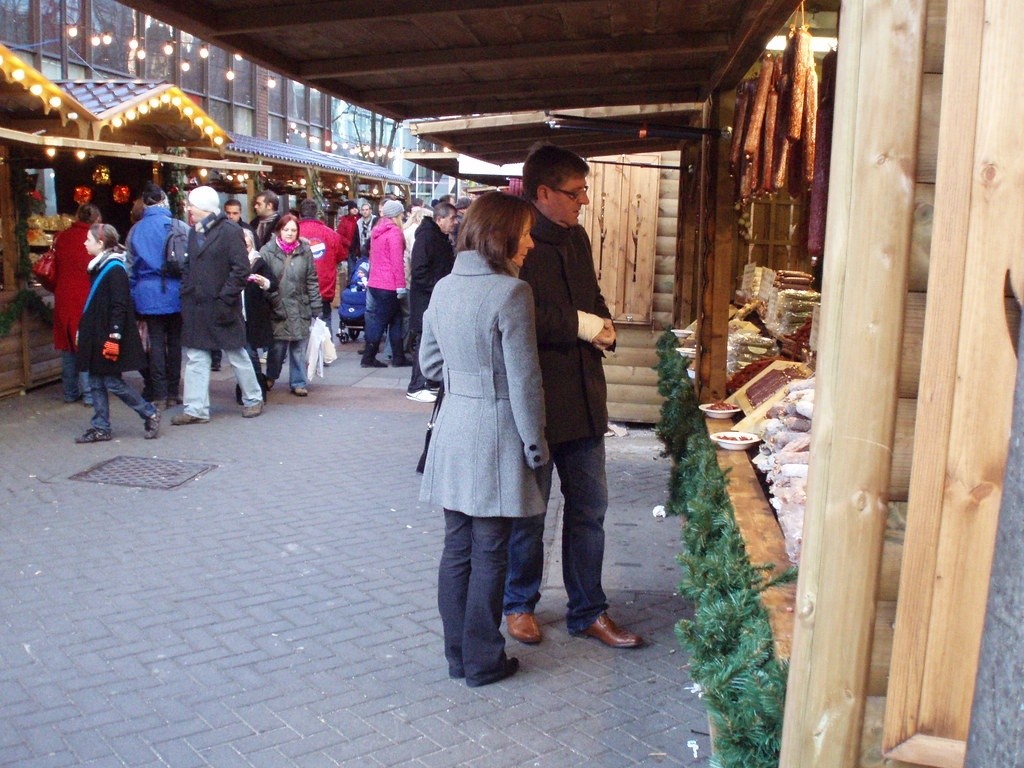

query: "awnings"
left=411, top=103, right=702, bottom=169
left=120, top=0, right=799, bottom=122
left=405, top=151, right=509, bottom=186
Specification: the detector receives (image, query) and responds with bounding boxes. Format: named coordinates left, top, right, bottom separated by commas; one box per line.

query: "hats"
left=358, top=262, right=370, bottom=275
left=455, top=197, right=472, bottom=208
left=348, top=201, right=357, bottom=210
left=383, top=201, right=405, bottom=217
left=189, top=186, right=221, bottom=216
left=141, top=182, right=169, bottom=208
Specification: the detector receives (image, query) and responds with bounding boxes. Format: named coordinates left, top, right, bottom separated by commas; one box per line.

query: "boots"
left=390, top=337, right=413, bottom=367
left=361, top=340, right=388, bottom=368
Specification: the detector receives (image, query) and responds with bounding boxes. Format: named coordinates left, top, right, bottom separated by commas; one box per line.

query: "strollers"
left=335, top=258, right=371, bottom=344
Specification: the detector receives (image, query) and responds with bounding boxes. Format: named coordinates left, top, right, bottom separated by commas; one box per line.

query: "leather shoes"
left=506, top=613, right=540, bottom=643
left=575, top=613, right=643, bottom=647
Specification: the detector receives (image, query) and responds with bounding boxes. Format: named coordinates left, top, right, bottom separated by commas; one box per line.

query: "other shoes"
left=242, top=403, right=263, bottom=418
left=266, top=377, right=274, bottom=388
left=154, top=398, right=177, bottom=411
left=291, top=387, right=309, bottom=396
left=171, top=413, right=209, bottom=424
left=503, top=657, right=519, bottom=675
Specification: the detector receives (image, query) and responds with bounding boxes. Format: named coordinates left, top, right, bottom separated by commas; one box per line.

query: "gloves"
left=103, top=338, right=120, bottom=361
left=396, top=288, right=407, bottom=299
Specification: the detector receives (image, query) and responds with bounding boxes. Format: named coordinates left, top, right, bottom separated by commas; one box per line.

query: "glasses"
left=552, top=186, right=589, bottom=201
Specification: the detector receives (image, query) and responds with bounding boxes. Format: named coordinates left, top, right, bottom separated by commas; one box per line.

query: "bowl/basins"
left=699, top=404, right=741, bottom=419
left=687, top=368, right=695, bottom=379
left=671, top=330, right=694, bottom=337
left=675, top=348, right=696, bottom=358
left=710, top=432, right=761, bottom=451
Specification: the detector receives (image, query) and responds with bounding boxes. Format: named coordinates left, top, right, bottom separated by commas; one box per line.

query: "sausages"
left=729, top=30, right=820, bottom=201
left=772, top=269, right=814, bottom=290
left=805, top=50, right=836, bottom=264
left=760, top=380, right=814, bottom=510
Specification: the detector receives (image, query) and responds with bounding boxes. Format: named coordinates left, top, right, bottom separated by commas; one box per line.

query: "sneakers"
left=144, top=409, right=160, bottom=438
left=75, top=428, right=111, bottom=443
left=406, top=387, right=439, bottom=403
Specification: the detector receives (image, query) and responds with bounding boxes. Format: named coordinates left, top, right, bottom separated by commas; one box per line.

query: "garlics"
left=734, top=203, right=751, bottom=242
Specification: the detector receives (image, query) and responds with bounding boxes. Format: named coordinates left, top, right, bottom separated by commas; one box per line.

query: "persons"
left=125, top=183, right=322, bottom=410
left=298, top=194, right=472, bottom=371
left=74, top=223, right=161, bottom=444
left=49, top=200, right=102, bottom=407
left=518, top=145, right=642, bottom=647
left=414, top=192, right=548, bottom=689
left=172, top=185, right=264, bottom=425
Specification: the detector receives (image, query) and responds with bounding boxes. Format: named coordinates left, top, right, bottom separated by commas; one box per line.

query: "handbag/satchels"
left=269, top=295, right=288, bottom=321
left=307, top=316, right=338, bottom=382
left=33, top=232, right=60, bottom=291
left=164, top=219, right=188, bottom=277
